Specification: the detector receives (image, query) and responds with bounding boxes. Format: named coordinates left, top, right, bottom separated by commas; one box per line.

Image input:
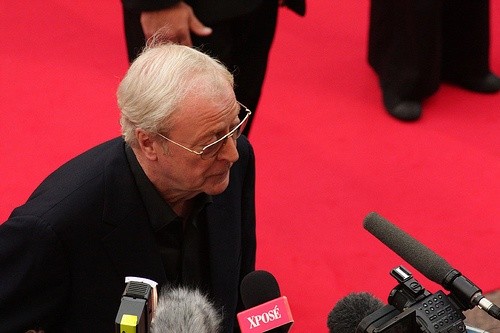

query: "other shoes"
left=380, top=74, right=422, bottom=120
left=443, top=69, right=500, bottom=93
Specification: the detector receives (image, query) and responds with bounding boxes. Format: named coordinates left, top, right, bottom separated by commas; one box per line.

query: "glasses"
left=155, top=101, right=252, bottom=160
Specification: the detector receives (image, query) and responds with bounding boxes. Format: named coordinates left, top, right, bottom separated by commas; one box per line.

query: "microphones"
left=236, top=270, right=294, bottom=333
left=151, top=288, right=221, bottom=333
left=115, top=277, right=158, bottom=333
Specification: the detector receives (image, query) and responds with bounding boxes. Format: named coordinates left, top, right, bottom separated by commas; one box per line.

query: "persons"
left=1, top=44, right=256, bottom=333
left=367, top=0, right=500, bottom=122
left=121, top=0, right=307, bottom=144
